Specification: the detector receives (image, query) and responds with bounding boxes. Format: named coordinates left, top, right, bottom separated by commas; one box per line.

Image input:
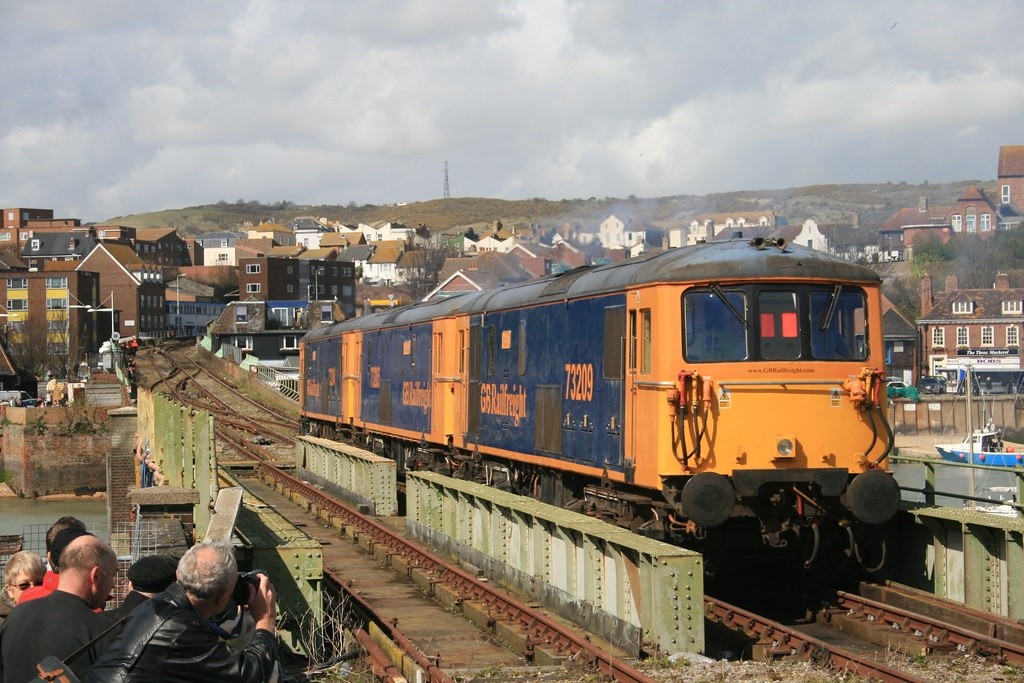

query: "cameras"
left=235, top=569, right=268, bottom=605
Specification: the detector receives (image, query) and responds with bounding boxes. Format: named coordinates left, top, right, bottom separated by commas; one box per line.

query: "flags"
left=959, top=369, right=966, bottom=381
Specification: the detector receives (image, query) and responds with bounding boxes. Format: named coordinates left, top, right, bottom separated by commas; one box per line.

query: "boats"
left=934, top=417, right=1024, bottom=466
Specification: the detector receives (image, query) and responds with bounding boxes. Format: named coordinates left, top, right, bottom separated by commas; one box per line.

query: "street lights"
left=87, top=307, right=115, bottom=336
left=176, top=274, right=186, bottom=314
left=67, top=304, right=91, bottom=351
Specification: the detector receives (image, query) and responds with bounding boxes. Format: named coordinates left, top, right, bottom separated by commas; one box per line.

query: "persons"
left=6, top=396, right=27, bottom=407
left=120, top=334, right=143, bottom=357
left=125, top=357, right=139, bottom=381
left=33, top=391, right=67, bottom=408
left=0, top=516, right=277, bottom=683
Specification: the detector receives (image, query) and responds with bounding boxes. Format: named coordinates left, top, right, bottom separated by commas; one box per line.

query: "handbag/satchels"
left=28, top=656, right=80, bottom=683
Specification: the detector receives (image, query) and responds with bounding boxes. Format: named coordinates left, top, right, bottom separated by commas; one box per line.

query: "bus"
left=970, top=369, right=1024, bottom=394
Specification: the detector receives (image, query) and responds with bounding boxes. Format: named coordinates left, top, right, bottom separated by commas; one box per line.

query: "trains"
left=297, top=231, right=902, bottom=568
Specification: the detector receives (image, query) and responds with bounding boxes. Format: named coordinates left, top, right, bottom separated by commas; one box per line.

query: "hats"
left=50, top=528, right=95, bottom=567
left=127, top=555, right=178, bottom=593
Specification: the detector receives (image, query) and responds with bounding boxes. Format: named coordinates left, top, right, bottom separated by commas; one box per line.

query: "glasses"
left=10, top=582, right=43, bottom=591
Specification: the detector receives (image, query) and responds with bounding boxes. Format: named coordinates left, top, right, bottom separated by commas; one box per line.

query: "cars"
left=888, top=375, right=946, bottom=394
